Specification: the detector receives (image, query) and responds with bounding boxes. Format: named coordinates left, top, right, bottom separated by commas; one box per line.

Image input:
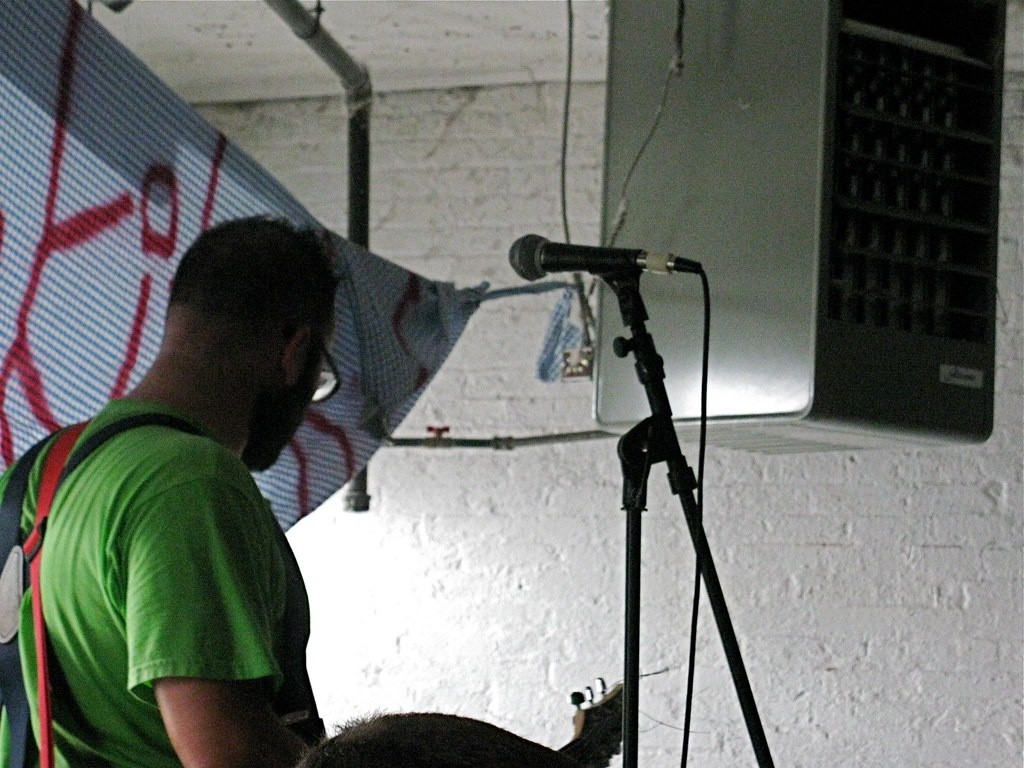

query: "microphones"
left=508, top=235, right=701, bottom=280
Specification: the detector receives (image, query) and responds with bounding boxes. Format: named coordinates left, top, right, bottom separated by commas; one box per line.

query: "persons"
left=0, top=213, right=335, bottom=768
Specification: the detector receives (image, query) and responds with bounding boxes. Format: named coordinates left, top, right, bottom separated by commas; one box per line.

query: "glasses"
left=310, top=327, right=341, bottom=404
